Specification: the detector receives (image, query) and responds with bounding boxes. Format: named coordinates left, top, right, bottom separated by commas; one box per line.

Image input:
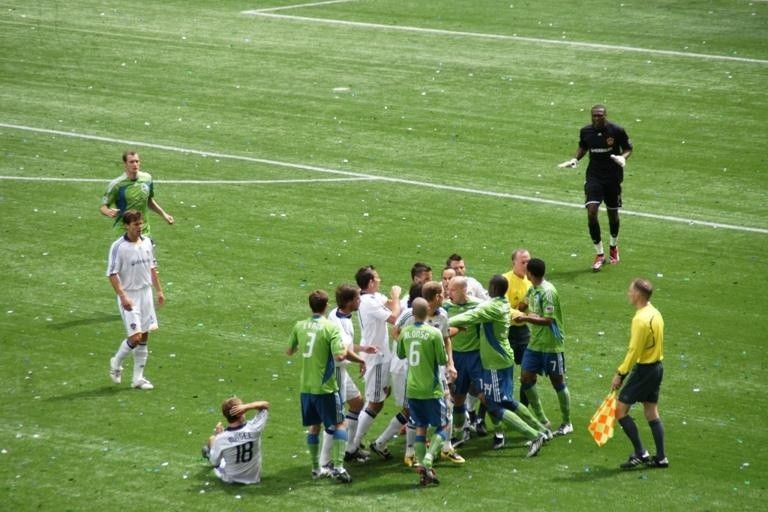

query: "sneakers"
left=527, top=431, right=549, bottom=457
left=523, top=420, right=553, bottom=447
left=491, top=432, right=506, bottom=449
left=645, top=457, right=669, bottom=468
left=415, top=464, right=441, bottom=487
left=619, top=452, right=650, bottom=469
left=609, top=246, right=620, bottom=264
left=440, top=446, right=465, bottom=465
left=369, top=440, right=393, bottom=460
left=590, top=253, right=607, bottom=270
left=343, top=449, right=371, bottom=465
left=404, top=454, right=419, bottom=467
left=131, top=378, right=154, bottom=390
left=202, top=445, right=211, bottom=460
left=450, top=410, right=488, bottom=447
left=110, top=357, right=122, bottom=384
left=326, top=465, right=351, bottom=482
left=312, top=469, right=324, bottom=480
left=401, top=424, right=432, bottom=450
left=556, top=422, right=574, bottom=435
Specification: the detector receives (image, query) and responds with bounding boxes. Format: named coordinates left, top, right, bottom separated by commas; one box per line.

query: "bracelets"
left=617, top=371, right=622, bottom=376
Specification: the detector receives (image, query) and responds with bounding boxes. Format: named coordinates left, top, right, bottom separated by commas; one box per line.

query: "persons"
left=611, top=278, right=670, bottom=467
left=100, top=151, right=173, bottom=239
left=203, top=395, right=270, bottom=485
left=558, top=105, right=634, bottom=269
left=106, top=210, right=164, bottom=387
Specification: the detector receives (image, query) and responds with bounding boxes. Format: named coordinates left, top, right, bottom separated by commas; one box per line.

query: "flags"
left=588, top=392, right=616, bottom=447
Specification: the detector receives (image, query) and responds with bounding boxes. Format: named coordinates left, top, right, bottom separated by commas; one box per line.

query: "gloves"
left=558, top=157, right=579, bottom=168
left=611, top=154, right=626, bottom=168
